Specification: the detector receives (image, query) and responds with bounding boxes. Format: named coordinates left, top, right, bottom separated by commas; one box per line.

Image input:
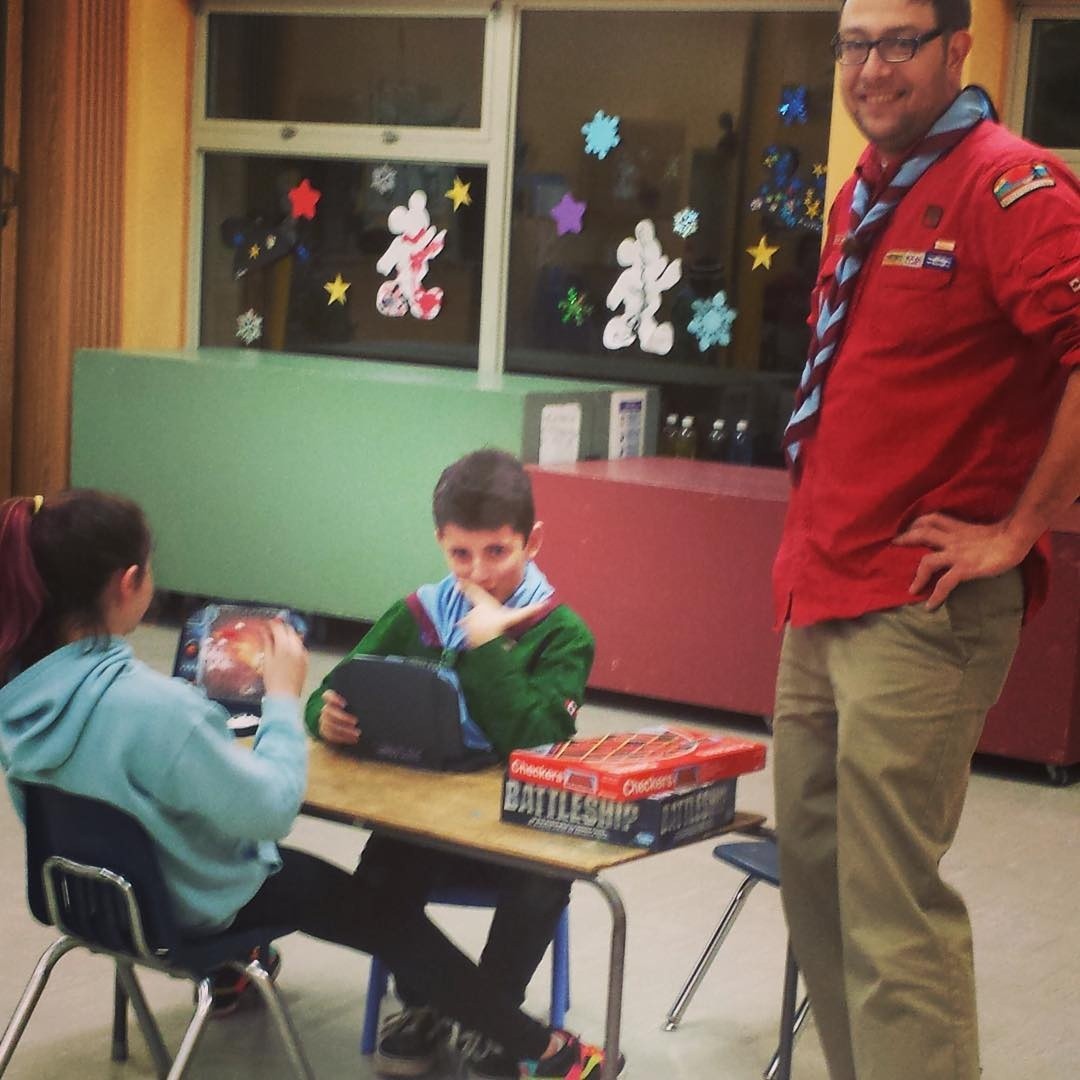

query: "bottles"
left=725, top=420, right=753, bottom=468
left=657, top=415, right=680, bottom=456
left=675, top=416, right=698, bottom=460
left=704, top=421, right=727, bottom=463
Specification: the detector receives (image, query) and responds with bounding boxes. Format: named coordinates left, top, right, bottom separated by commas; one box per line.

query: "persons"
left=769, top=1, right=1080, bottom=1080
left=304, top=447, right=595, bottom=1080
left=0, top=488, right=626, bottom=1080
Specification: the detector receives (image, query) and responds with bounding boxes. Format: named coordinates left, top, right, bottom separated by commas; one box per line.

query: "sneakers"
left=438, top=1021, right=522, bottom=1080
left=377, top=1003, right=445, bottom=1058
left=520, top=1027, right=625, bottom=1080
left=193, top=945, right=283, bottom=1017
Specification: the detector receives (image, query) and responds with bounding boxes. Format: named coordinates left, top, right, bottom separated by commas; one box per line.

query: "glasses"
left=829, top=25, right=947, bottom=66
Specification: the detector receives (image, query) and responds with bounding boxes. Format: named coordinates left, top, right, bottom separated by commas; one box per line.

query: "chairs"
left=662, top=834, right=820, bottom=1080
left=0, top=770, right=313, bottom=1080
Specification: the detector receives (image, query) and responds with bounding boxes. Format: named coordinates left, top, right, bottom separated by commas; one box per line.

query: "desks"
left=528, top=453, right=1080, bottom=790
left=216, top=724, right=791, bottom=1080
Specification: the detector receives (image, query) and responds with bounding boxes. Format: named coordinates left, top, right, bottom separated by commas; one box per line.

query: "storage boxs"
left=497, top=769, right=739, bottom=849
left=507, top=725, right=768, bottom=802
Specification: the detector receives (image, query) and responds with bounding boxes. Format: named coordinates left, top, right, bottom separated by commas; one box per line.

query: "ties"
left=781, top=85, right=991, bottom=478
